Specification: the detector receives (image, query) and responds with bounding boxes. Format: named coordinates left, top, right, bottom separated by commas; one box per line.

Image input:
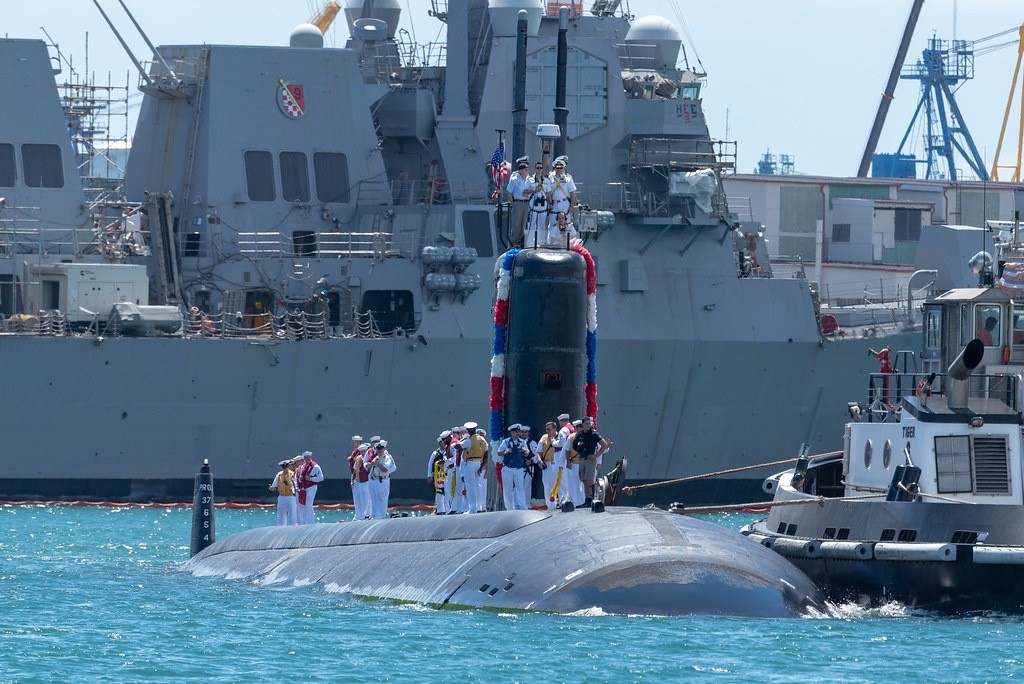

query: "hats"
left=518, top=163, right=528, bottom=171
left=277, top=413, right=595, bottom=468
left=552, top=160, right=566, bottom=168
left=516, top=155, right=530, bottom=165
left=555, top=154, right=569, bottom=164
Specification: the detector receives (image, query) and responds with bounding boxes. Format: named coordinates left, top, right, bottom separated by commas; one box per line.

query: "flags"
left=489, top=140, right=507, bottom=199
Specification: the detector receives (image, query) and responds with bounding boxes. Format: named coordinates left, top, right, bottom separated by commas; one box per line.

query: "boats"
left=0, top=0, right=1024, bottom=617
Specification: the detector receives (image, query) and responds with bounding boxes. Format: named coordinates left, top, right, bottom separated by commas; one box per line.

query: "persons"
left=535, top=414, right=614, bottom=511
left=497, top=423, right=538, bottom=511
left=347, top=435, right=396, bottom=521
left=428, top=422, right=489, bottom=515
left=1014, top=315, right=1024, bottom=345
left=976, top=317, right=997, bottom=346
left=269, top=452, right=324, bottom=526
left=506, top=155, right=577, bottom=247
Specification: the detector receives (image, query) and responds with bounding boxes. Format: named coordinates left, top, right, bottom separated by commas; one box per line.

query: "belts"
left=514, top=199, right=529, bottom=202
left=556, top=198, right=566, bottom=203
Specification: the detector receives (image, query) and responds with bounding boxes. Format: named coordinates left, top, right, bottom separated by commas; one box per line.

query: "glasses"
left=545, top=427, right=553, bottom=431
left=536, top=167, right=543, bottom=169
